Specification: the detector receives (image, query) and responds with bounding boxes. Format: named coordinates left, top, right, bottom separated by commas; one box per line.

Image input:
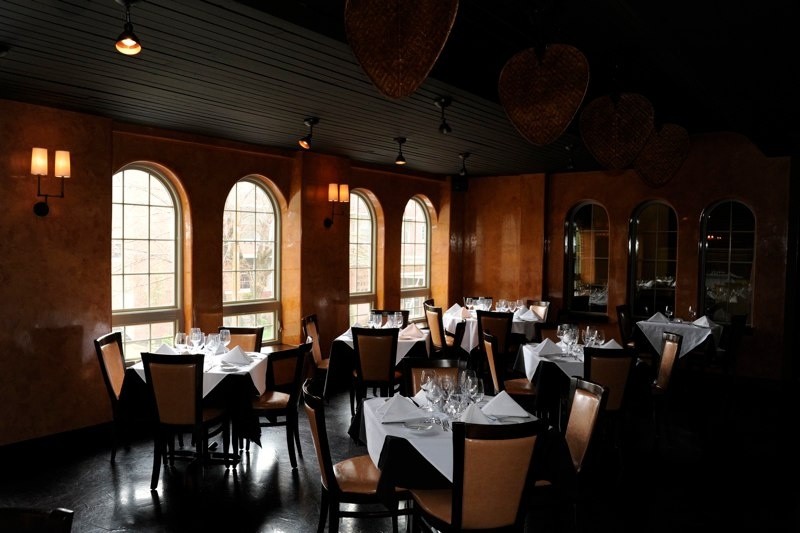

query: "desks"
left=518, top=340, right=603, bottom=394
left=359, top=388, right=556, bottom=533
left=323, top=320, right=430, bottom=416
left=634, top=315, right=724, bottom=379
left=444, top=306, right=540, bottom=352
left=126, top=347, right=268, bottom=468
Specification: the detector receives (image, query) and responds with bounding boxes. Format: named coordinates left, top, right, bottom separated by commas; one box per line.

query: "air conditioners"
left=392, top=136, right=408, bottom=165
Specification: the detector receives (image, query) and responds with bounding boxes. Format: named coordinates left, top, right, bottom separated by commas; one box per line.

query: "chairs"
left=92, top=300, right=683, bottom=533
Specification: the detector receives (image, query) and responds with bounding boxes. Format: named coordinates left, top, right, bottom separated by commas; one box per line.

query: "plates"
left=403, top=420, right=436, bottom=433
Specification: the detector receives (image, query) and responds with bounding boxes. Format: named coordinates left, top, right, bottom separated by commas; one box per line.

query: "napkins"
left=647, top=310, right=669, bottom=322
left=533, top=336, right=563, bottom=356
left=693, top=316, right=716, bottom=327
left=446, top=303, right=462, bottom=315
left=600, top=337, right=623, bottom=349
left=454, top=307, right=471, bottom=318
left=513, top=304, right=528, bottom=319
left=399, top=322, right=424, bottom=338
left=517, top=309, right=540, bottom=321
left=374, top=391, right=431, bottom=423
left=153, top=343, right=177, bottom=354
left=481, top=391, right=532, bottom=419
left=458, top=402, right=492, bottom=425
left=220, top=345, right=254, bottom=365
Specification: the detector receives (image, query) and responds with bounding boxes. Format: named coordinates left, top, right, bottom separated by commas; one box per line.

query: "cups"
left=495, top=302, right=502, bottom=312
left=373, top=315, right=382, bottom=328
left=388, top=315, right=394, bottom=328
left=394, top=312, right=403, bottom=328
left=479, top=297, right=485, bottom=304
left=499, top=299, right=506, bottom=309
left=508, top=302, right=516, bottom=312
left=674, top=319, right=683, bottom=324
left=586, top=326, right=598, bottom=340
left=516, top=300, right=523, bottom=308
left=472, top=301, right=479, bottom=309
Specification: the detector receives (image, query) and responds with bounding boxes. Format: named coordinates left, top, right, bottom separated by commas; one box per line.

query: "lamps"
left=323, top=183, right=350, bottom=227
left=457, top=152, right=472, bottom=177
left=434, top=97, right=452, bottom=135
left=27, top=147, right=72, bottom=217
left=298, top=117, right=320, bottom=150
left=114, top=0, right=143, bottom=57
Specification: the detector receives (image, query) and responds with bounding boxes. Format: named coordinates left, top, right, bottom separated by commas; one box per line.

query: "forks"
left=442, top=421, right=449, bottom=431
left=485, top=415, right=531, bottom=423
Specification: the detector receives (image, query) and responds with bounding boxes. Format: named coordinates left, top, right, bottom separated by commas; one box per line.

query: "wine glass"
left=439, top=369, right=485, bottom=421
left=557, top=324, right=569, bottom=348
left=367, top=313, right=374, bottom=328
left=688, top=306, right=696, bottom=324
left=426, top=376, right=442, bottom=414
left=563, top=328, right=579, bottom=356
left=595, top=330, right=605, bottom=348
left=466, top=298, right=474, bottom=314
left=666, top=306, right=673, bottom=323
left=581, top=330, right=592, bottom=347
left=420, top=370, right=435, bottom=409
left=485, top=299, right=492, bottom=311
left=175, top=328, right=231, bottom=355
